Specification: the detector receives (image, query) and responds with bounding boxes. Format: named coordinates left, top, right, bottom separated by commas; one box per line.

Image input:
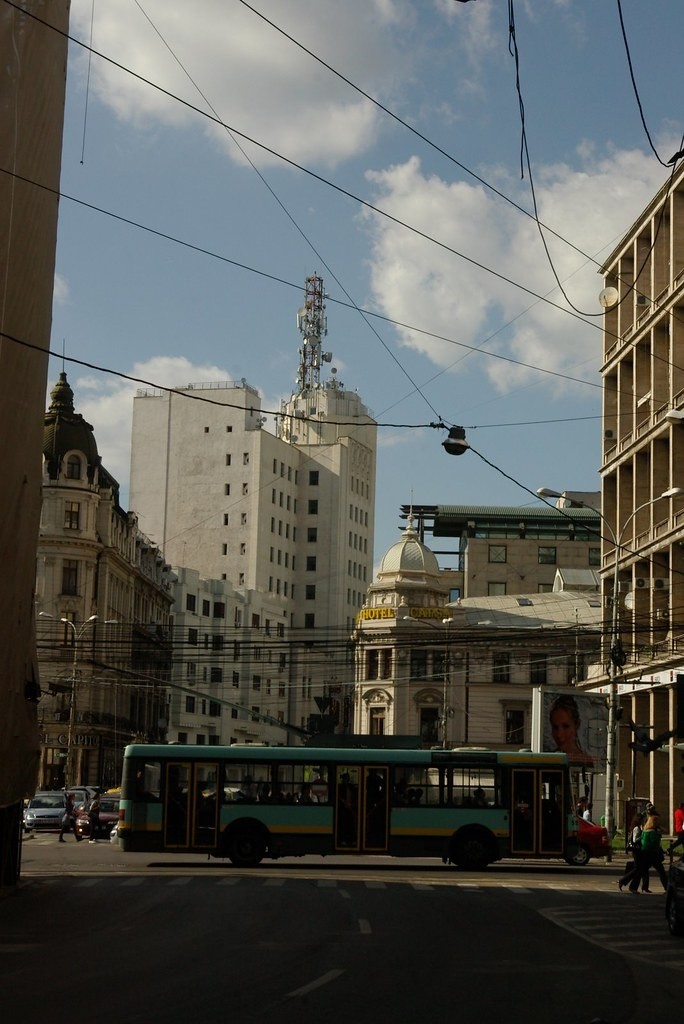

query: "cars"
left=25, top=785, right=120, bottom=838
left=564, top=815, right=607, bottom=865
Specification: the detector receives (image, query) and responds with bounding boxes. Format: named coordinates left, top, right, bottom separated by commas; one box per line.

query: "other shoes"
left=642, top=887, right=652, bottom=893
left=617, top=880, right=623, bottom=892
left=629, top=887, right=641, bottom=894
left=89, top=841, right=97, bottom=844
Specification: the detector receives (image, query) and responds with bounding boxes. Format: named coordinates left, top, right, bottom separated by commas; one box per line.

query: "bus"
left=120, top=739, right=573, bottom=872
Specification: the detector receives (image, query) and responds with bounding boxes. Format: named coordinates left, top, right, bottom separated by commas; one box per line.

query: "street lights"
left=538, top=487, right=683, bottom=864
left=40, top=611, right=117, bottom=790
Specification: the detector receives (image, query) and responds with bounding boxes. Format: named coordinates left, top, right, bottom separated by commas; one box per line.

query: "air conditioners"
left=635, top=578, right=650, bottom=589
left=605, top=596, right=614, bottom=608
left=636, top=295, right=650, bottom=306
left=652, top=578, right=670, bottom=591
left=657, top=609, right=670, bottom=621
left=604, top=430, right=617, bottom=439
left=618, top=582, right=632, bottom=593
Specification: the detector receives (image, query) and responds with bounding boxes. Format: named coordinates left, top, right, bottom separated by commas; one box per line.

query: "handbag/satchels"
left=626, top=825, right=642, bottom=851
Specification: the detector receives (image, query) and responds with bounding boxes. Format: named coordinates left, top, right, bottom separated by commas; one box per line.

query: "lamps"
left=441, top=426, right=470, bottom=456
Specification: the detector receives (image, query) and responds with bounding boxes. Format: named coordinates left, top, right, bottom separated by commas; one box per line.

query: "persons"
left=338, top=768, right=385, bottom=848
left=617, top=802, right=684, bottom=895
left=548, top=694, right=593, bottom=768
left=223, top=772, right=316, bottom=804
left=58, top=791, right=85, bottom=843
left=576, top=796, right=592, bottom=821
left=471, top=788, right=489, bottom=805
left=87, top=793, right=101, bottom=844
left=168, top=769, right=217, bottom=847
left=401, top=772, right=425, bottom=800
left=136, top=764, right=158, bottom=798
